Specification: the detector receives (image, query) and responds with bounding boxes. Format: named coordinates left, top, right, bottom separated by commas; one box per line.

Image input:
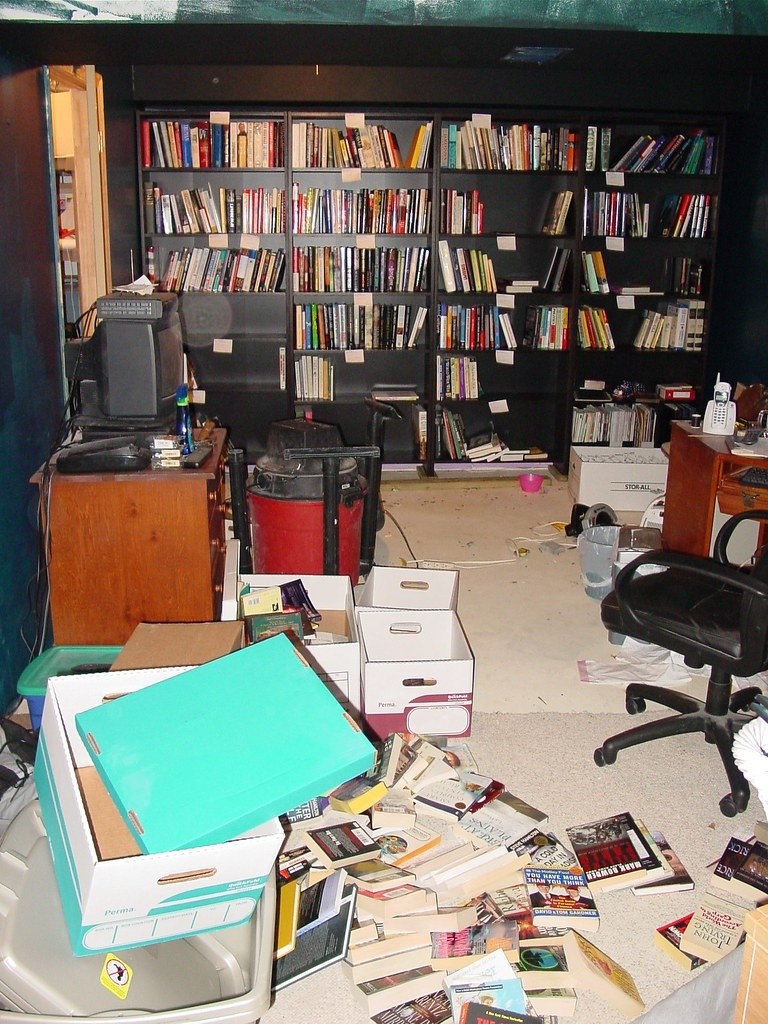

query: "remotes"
left=743, top=431, right=758, bottom=445
left=183, top=447, right=214, bottom=469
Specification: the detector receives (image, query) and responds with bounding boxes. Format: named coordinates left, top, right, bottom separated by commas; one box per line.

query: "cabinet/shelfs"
left=133, top=103, right=727, bottom=481
left=30, top=426, right=227, bottom=648
left=53, top=155, right=83, bottom=339
left=661, top=420, right=768, bottom=571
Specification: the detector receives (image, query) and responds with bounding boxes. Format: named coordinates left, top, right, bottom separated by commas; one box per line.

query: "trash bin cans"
left=576, top=525, right=625, bottom=601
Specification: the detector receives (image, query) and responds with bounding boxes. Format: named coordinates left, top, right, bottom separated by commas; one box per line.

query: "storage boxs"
left=732, top=902, right=768, bottom=1024
left=0, top=567, right=474, bottom=1024
left=567, top=445, right=670, bottom=512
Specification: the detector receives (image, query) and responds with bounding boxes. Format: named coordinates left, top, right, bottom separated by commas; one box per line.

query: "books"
left=271, top=732, right=768, bottom=1024
left=236, top=578, right=349, bottom=645
left=141, top=120, right=715, bottom=462
left=725, top=436, right=768, bottom=457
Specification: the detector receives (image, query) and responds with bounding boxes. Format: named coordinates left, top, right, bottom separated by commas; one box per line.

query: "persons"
left=565, top=886, right=596, bottom=909
left=443, top=750, right=460, bottom=766
left=530, top=884, right=559, bottom=908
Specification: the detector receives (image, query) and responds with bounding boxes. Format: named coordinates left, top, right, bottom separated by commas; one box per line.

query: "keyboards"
left=738, top=467, right=768, bottom=488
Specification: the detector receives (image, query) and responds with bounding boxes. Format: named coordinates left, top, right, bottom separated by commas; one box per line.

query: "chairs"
left=594, top=509, right=768, bottom=818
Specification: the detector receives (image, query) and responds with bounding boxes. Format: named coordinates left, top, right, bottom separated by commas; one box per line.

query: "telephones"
left=703, top=371, right=736, bottom=434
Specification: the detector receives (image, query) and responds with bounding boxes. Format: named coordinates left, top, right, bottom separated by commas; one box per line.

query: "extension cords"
left=422, top=560, right=455, bottom=571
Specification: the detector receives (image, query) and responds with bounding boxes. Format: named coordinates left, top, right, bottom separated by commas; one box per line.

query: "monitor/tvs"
left=64, top=315, right=183, bottom=423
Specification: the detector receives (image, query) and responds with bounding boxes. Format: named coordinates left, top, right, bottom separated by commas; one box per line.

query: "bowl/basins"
left=518, top=473, right=544, bottom=492
